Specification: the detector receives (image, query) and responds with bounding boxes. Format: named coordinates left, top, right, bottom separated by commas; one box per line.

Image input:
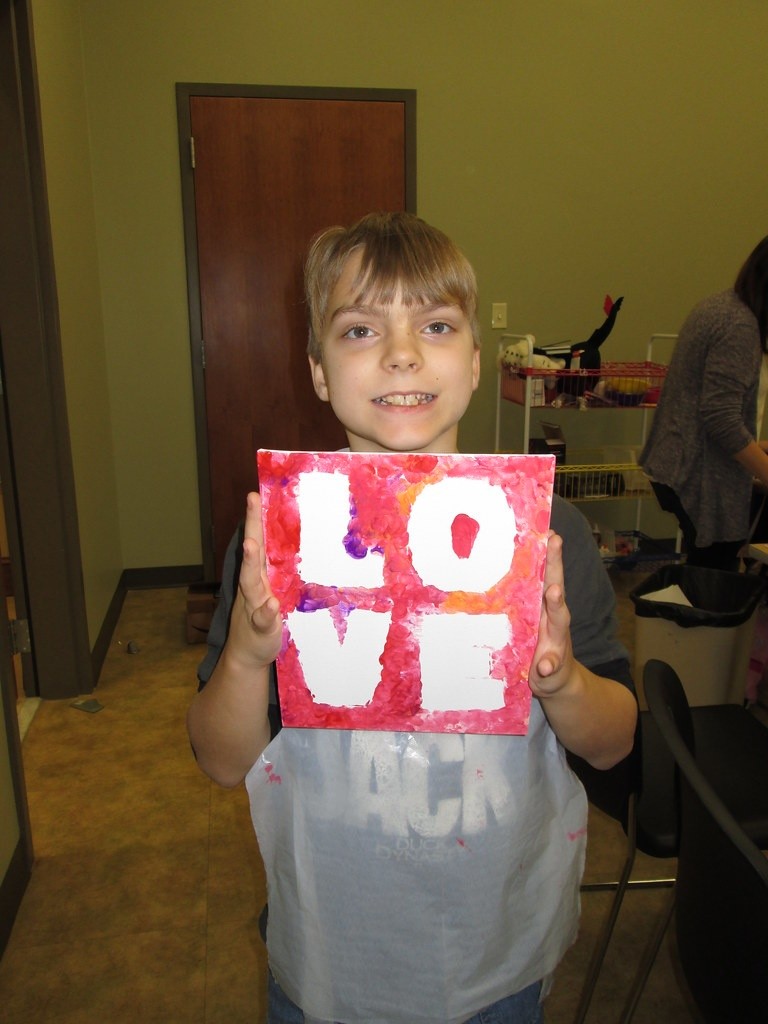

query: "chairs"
left=571, top=654, right=767, bottom=1022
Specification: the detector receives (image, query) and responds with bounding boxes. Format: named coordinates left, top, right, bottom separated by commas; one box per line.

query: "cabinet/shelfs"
left=496, top=331, right=695, bottom=569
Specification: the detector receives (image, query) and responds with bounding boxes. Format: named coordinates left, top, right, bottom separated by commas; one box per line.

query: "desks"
left=748, top=540, right=766, bottom=571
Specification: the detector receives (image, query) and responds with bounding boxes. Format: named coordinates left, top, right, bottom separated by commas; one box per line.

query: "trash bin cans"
left=630, top=563, right=762, bottom=711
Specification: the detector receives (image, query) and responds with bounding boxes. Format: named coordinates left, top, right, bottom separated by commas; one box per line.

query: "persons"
left=187, top=214, right=638, bottom=1024
left=635, top=234, right=768, bottom=562
